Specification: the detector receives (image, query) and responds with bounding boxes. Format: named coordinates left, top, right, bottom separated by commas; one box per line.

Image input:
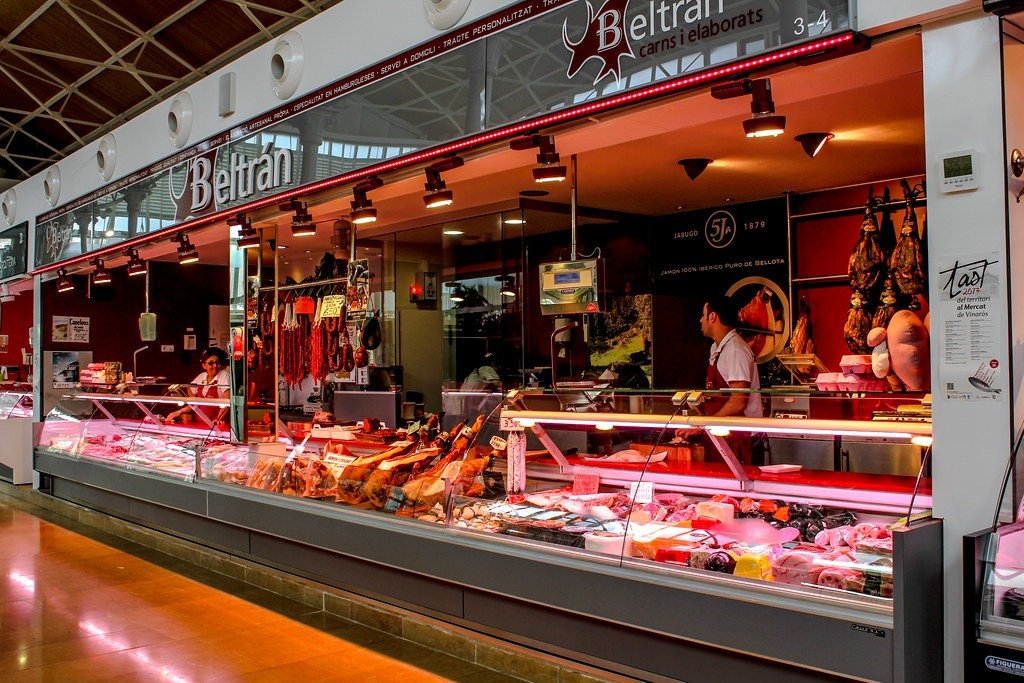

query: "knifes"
left=543, top=448, right=578, bottom=457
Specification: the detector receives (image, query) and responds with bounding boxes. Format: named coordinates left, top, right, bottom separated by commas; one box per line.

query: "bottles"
left=443, top=376, right=455, bottom=389
left=527, top=378, right=543, bottom=387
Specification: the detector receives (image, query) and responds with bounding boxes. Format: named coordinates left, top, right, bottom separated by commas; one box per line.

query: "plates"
left=758, top=464, right=802, bottom=473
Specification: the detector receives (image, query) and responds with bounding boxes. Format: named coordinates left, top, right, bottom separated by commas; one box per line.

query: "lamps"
left=494, top=274, right=517, bottom=296
left=349, top=177, right=384, bottom=225
left=89, top=256, right=112, bottom=284
left=278, top=197, right=316, bottom=236
left=794, top=132, right=835, bottom=160
left=121, top=247, right=147, bottom=276
left=445, top=280, right=465, bottom=302
left=710, top=78, right=786, bottom=138
left=226, top=212, right=258, bottom=249
left=508, top=131, right=567, bottom=185
left=56, top=266, right=75, bottom=292
left=678, top=158, right=714, bottom=181
left=422, top=157, right=463, bottom=208
left=170, top=231, right=200, bottom=266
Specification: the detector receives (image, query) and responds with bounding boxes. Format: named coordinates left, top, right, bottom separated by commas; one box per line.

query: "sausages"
left=248, top=306, right=354, bottom=390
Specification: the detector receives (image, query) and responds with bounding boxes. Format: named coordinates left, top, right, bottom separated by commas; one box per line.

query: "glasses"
left=202, top=361, right=220, bottom=365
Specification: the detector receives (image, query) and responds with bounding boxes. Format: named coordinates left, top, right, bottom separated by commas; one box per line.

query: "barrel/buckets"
left=401, top=401, right=425, bottom=419
left=258, top=442, right=287, bottom=464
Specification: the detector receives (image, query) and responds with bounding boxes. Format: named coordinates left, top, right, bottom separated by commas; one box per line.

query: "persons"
left=675, top=297, right=764, bottom=466
left=462, top=352, right=507, bottom=392
left=166, top=347, right=230, bottom=427
